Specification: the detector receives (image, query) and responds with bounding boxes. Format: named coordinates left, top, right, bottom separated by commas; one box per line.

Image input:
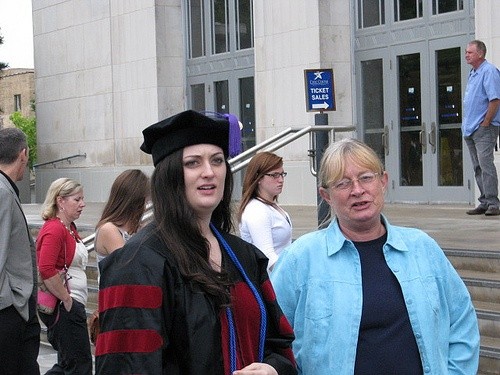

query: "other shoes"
left=466, top=204, right=486, bottom=215
left=485, top=207, right=499, bottom=216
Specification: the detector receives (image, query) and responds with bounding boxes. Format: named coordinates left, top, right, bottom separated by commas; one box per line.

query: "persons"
left=270, top=137, right=481, bottom=375
left=461, top=40, right=500, bottom=215
left=237, top=151, right=293, bottom=276
left=0, top=127, right=41, bottom=375
left=93, top=169, right=150, bottom=287
left=94, top=110, right=298, bottom=375
left=36, top=177, right=93, bottom=375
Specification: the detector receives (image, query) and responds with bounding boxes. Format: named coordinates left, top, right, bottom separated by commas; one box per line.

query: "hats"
left=140, top=110, right=240, bottom=168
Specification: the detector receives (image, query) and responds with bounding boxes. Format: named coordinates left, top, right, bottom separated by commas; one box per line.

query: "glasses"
left=328, top=172, right=378, bottom=191
left=263, top=173, right=287, bottom=178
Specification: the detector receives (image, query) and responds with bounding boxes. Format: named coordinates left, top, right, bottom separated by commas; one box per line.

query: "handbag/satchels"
left=88, top=309, right=99, bottom=346
left=37, top=264, right=71, bottom=331
